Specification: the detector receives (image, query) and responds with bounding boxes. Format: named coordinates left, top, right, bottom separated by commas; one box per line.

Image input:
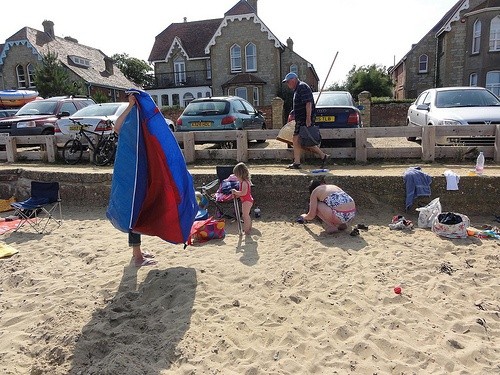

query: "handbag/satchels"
left=430, top=211, right=470, bottom=239
left=299, top=125, right=320, bottom=146
left=415, top=197, right=442, bottom=228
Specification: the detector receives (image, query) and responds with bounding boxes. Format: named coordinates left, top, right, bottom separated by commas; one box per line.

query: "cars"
left=175, top=95, right=267, bottom=149
left=54, top=102, right=175, bottom=148
left=406, top=86, right=500, bottom=146
left=287, top=90, right=363, bottom=147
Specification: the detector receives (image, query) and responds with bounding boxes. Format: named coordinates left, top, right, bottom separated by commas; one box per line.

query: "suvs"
left=0, top=89, right=98, bottom=151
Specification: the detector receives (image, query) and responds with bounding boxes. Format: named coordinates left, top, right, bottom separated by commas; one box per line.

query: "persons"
left=232, top=163, right=255, bottom=235
left=282, top=73, right=330, bottom=169
left=300, top=177, right=356, bottom=233
left=113, top=89, right=158, bottom=264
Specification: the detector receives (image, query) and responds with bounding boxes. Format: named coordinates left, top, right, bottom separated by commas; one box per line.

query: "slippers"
left=136, top=258, right=156, bottom=267
left=131, top=253, right=153, bottom=258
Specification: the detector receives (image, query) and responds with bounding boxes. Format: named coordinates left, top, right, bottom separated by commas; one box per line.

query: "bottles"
left=89, top=136, right=97, bottom=145
left=254, top=202, right=260, bottom=217
left=475, top=151, right=484, bottom=175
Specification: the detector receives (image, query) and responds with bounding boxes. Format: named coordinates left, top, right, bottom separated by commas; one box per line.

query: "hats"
left=282, top=72, right=299, bottom=84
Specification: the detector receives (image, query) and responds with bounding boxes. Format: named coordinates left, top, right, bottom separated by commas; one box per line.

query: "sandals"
left=285, top=162, right=302, bottom=169
left=322, top=154, right=331, bottom=168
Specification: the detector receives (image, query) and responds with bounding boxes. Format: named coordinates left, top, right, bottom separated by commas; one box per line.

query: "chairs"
left=205, top=103, right=215, bottom=111
left=10, top=180, right=62, bottom=234
left=195, top=165, right=257, bottom=237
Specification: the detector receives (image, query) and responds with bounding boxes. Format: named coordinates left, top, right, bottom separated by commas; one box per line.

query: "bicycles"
left=62, top=115, right=118, bottom=166
